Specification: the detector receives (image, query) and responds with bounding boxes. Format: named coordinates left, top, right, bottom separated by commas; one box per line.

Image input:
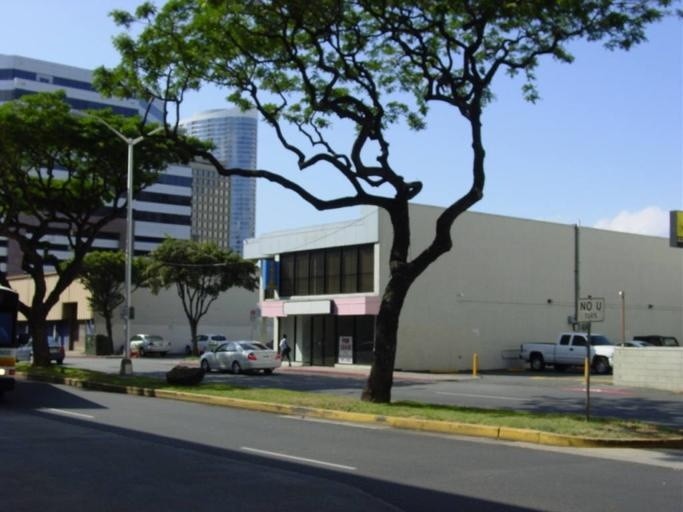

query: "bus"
left=0, top=285, right=19, bottom=378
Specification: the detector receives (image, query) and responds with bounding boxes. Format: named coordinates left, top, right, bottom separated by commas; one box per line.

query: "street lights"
left=69, top=107, right=185, bottom=377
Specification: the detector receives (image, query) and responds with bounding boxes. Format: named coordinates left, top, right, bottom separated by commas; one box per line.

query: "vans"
left=633, top=337, right=680, bottom=346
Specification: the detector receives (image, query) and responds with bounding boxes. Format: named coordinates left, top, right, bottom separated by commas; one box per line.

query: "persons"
left=278, top=334, right=292, bottom=366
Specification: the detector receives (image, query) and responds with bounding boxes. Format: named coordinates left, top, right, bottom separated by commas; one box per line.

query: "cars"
left=185, top=334, right=227, bottom=356
left=16, top=336, right=65, bottom=364
left=129, top=334, right=170, bottom=357
left=616, top=340, right=654, bottom=347
left=201, top=341, right=281, bottom=374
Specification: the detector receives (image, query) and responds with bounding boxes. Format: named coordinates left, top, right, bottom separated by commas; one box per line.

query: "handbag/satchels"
left=285, top=347, right=291, bottom=353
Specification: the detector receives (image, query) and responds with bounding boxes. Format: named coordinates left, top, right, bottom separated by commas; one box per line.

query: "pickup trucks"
left=520, top=333, right=621, bottom=374
